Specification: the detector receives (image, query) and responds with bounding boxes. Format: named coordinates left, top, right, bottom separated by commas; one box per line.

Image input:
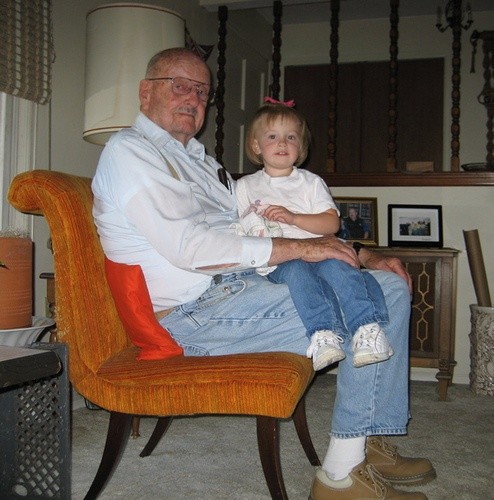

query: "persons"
left=91, top=47, right=436, bottom=500
left=408, top=221, right=429, bottom=235
left=338, top=207, right=369, bottom=239
left=237, top=96, right=393, bottom=371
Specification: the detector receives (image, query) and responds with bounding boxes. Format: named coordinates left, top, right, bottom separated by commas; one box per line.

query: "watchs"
left=353, top=241, right=365, bottom=254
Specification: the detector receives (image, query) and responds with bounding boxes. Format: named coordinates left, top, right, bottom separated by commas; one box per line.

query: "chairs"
left=7, top=170, right=322, bottom=500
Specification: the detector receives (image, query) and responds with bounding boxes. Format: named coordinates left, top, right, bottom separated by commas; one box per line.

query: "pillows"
left=104, top=255, right=184, bottom=363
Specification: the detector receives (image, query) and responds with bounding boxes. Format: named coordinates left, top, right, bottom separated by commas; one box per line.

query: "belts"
left=154, top=307, right=175, bottom=321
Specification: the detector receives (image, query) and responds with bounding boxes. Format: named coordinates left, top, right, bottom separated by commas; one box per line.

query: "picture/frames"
left=333, top=196, right=378, bottom=246
left=387, top=204, right=444, bottom=248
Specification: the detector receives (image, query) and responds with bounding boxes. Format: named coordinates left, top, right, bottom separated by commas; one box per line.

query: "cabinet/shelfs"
left=370, top=247, right=462, bottom=401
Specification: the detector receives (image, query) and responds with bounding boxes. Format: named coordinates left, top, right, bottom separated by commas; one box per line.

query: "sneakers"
left=308, top=461, right=427, bottom=500
left=306, top=330, right=344, bottom=371
left=366, top=435, right=436, bottom=486
left=352, top=323, right=393, bottom=367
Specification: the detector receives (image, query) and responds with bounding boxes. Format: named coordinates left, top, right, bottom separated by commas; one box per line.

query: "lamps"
left=83, top=3, right=186, bottom=148
left=434, top=1, right=474, bottom=34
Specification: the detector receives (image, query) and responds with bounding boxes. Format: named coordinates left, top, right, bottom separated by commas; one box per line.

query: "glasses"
left=149, top=77, right=216, bottom=103
